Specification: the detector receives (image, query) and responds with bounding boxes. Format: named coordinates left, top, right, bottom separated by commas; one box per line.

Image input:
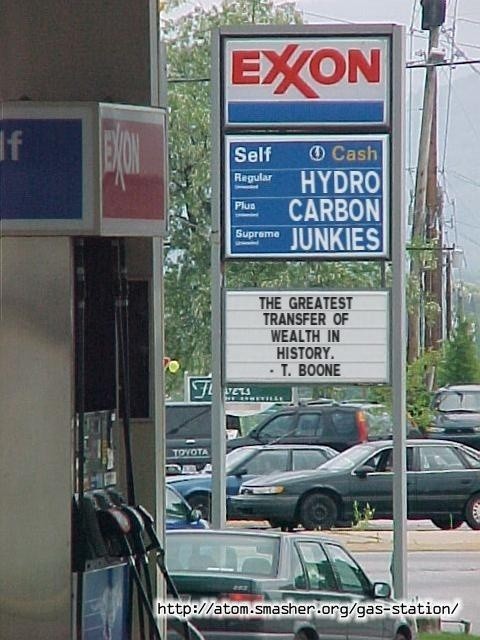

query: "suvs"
left=226, top=399, right=425, bottom=454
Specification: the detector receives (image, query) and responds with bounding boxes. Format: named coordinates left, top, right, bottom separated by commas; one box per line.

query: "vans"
left=165, top=401, right=211, bottom=475
left=425, top=383, right=480, bottom=450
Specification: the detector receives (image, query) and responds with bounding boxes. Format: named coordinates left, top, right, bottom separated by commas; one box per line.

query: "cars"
left=165, top=529, right=416, bottom=640
